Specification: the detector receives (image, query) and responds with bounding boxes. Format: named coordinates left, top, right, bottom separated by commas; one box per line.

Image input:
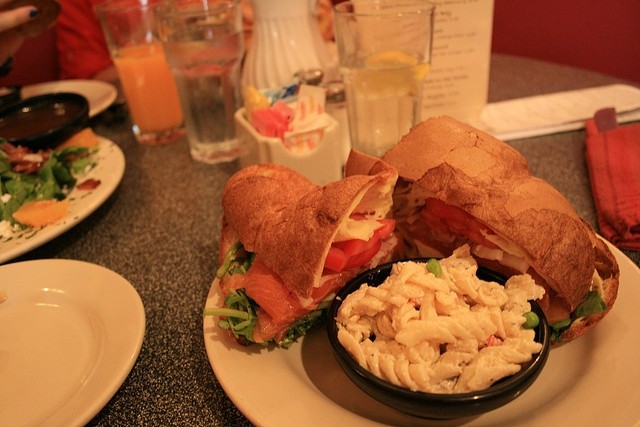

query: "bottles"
left=240, top=0, right=328, bottom=110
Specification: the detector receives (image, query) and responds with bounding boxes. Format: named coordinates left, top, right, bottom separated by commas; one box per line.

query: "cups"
left=330, top=0, right=436, bottom=158
left=95, top=0, right=186, bottom=147
left=152, top=1, right=245, bottom=165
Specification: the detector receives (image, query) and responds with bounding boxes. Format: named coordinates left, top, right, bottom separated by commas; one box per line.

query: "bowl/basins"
left=326, top=257, right=551, bottom=421
left=0, top=92, right=89, bottom=153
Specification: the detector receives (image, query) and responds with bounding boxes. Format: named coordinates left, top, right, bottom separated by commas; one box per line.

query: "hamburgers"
left=369, top=115, right=620, bottom=350
left=204, top=147, right=398, bottom=350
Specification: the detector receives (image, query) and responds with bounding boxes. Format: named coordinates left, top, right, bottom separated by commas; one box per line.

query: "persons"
left=56, top=1, right=254, bottom=85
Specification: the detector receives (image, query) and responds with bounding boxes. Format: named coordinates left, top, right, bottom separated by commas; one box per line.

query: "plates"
left=201, top=233, right=640, bottom=426
left=19, top=79, right=118, bottom=119
left=0, top=258, right=146, bottom=427
left=0, top=135, right=126, bottom=264
